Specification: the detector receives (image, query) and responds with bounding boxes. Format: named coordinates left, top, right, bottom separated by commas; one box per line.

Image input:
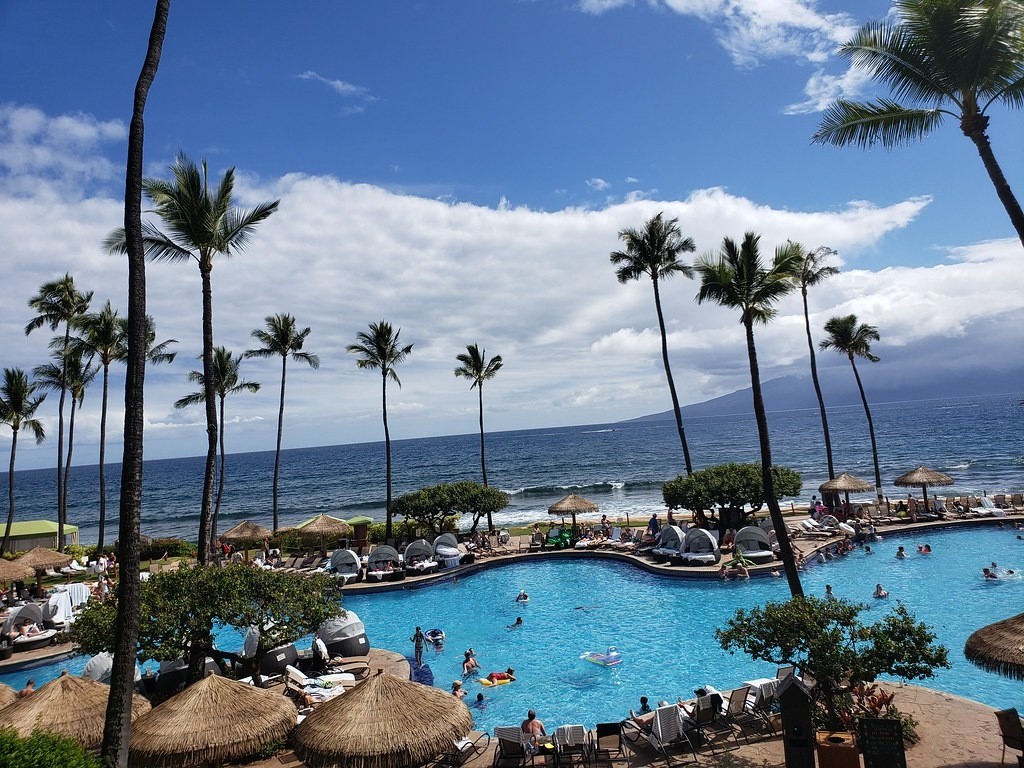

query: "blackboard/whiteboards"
left=860, top=719, right=907, bottom=768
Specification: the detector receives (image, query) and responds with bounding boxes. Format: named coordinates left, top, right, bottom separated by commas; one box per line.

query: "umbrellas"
left=894, top=465, right=956, bottom=512
left=298, top=671, right=474, bottom=768
left=818, top=473, right=874, bottom=508
left=548, top=493, right=599, bottom=527
left=963, top=612, right=1024, bottom=682
left=0, top=675, right=152, bottom=748
left=128, top=670, right=298, bottom=768
left=300, top=514, right=348, bottom=558
left=222, top=520, right=273, bottom=562
left=11, top=546, right=73, bottom=597
left=0, top=556, right=36, bottom=590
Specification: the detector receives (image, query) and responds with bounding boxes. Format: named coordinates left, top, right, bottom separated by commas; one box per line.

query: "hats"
left=694, top=688, right=706, bottom=696
left=652, top=513, right=657, bottom=516
left=659, top=700, right=669, bottom=707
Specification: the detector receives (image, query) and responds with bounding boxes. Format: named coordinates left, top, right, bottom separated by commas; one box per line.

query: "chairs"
left=38, top=554, right=120, bottom=580
left=465, top=528, right=645, bottom=559
left=438, top=666, right=850, bottom=768
left=150, top=562, right=179, bottom=573
left=239, top=639, right=372, bottom=711
left=708, top=516, right=840, bottom=549
left=945, top=493, right=1024, bottom=517
left=253, top=551, right=324, bottom=574
left=991, top=703, right=1024, bottom=768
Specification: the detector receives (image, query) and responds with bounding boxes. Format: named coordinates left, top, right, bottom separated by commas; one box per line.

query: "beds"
left=849, top=501, right=938, bottom=526
left=1, top=573, right=223, bottom=695
left=431, top=533, right=464, bottom=568
left=366, top=543, right=406, bottom=582
left=313, top=608, right=370, bottom=656
left=245, top=621, right=298, bottom=667
left=328, top=549, right=364, bottom=584
left=682, top=529, right=722, bottom=565
left=403, top=539, right=438, bottom=575
left=734, top=528, right=775, bottom=563
left=652, top=526, right=686, bottom=562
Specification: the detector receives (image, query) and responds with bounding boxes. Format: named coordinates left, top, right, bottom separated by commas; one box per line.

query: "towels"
left=562, top=724, right=576, bottom=747
left=315, top=638, right=328, bottom=660
left=304, top=684, right=345, bottom=702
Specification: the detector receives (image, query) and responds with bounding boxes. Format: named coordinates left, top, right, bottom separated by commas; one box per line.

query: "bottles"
left=535, top=742, right=539, bottom=753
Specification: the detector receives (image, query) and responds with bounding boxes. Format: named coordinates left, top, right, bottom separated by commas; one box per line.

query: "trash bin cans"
left=0, top=635, right=14, bottom=660
left=815, top=728, right=861, bottom=768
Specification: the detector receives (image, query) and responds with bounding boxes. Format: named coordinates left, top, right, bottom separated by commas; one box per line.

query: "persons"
left=657, top=700, right=668, bottom=708
left=677, top=689, right=707, bottom=717
left=298, top=689, right=316, bottom=709
left=473, top=692, right=488, bottom=710
left=0, top=493, right=1024, bottom=698
left=59, top=670, right=69, bottom=677
left=633, top=697, right=652, bottom=717
left=520, top=709, right=547, bottom=737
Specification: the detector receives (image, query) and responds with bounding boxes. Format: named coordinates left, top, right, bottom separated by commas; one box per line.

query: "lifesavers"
left=424, top=628, right=445, bottom=642
left=819, top=515, right=839, bottom=535
left=838, top=522, right=855, bottom=534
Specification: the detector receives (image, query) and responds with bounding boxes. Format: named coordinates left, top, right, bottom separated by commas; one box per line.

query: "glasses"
left=25, top=621, right=30, bottom=623
